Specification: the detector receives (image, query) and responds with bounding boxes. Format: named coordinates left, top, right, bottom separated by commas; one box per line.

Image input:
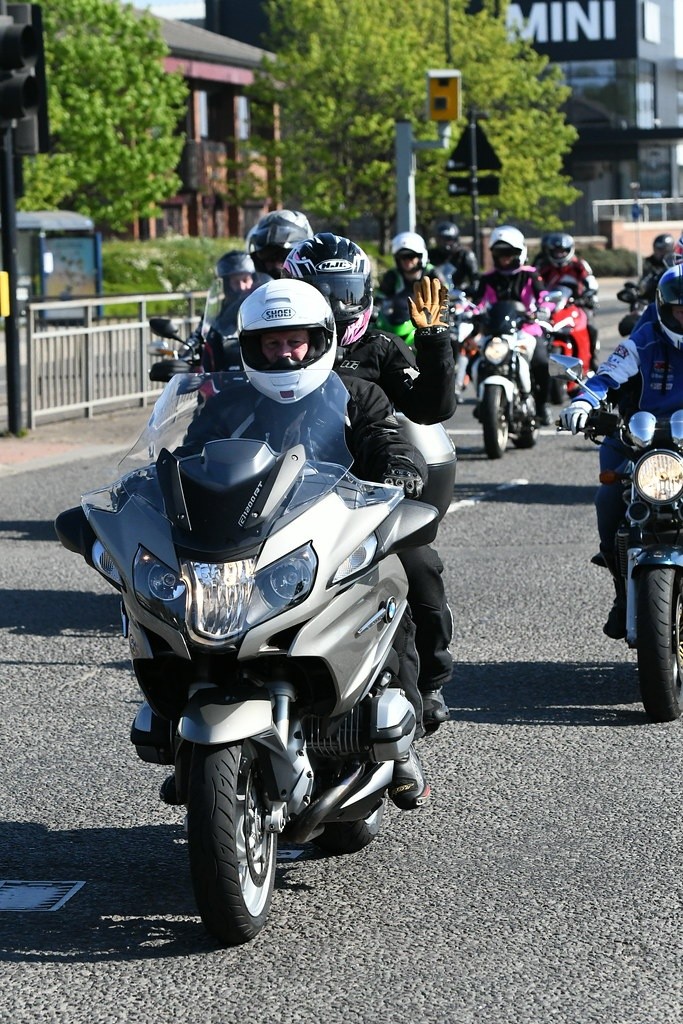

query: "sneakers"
left=422, top=686, right=450, bottom=726
left=388, top=743, right=430, bottom=810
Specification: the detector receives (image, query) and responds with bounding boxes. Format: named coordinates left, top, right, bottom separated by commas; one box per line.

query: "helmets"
left=215, top=249, right=255, bottom=278
left=654, top=234, right=675, bottom=263
left=545, top=232, right=576, bottom=268
left=488, top=225, right=528, bottom=266
left=655, top=264, right=683, bottom=352
left=236, top=278, right=338, bottom=405
left=280, top=233, right=374, bottom=346
left=245, top=210, right=314, bottom=256
left=391, top=232, right=428, bottom=268
left=434, top=222, right=462, bottom=254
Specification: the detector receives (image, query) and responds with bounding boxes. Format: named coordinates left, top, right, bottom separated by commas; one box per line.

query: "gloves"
left=560, top=401, right=592, bottom=435
left=537, top=307, right=550, bottom=321
left=380, top=468, right=425, bottom=499
left=407, top=276, right=450, bottom=328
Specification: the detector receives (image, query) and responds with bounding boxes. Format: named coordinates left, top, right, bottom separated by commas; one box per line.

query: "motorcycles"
left=145, top=279, right=646, bottom=460
left=52, top=367, right=451, bottom=941
left=548, top=352, right=683, bottom=721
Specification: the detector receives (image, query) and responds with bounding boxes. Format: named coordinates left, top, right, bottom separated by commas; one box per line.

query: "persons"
left=560, top=229, right=683, bottom=639
left=432, top=223, right=600, bottom=426
left=111, top=233, right=456, bottom=810
left=248, top=211, right=314, bottom=280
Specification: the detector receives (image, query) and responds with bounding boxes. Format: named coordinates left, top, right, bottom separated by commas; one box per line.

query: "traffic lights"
left=0, top=14, right=43, bottom=130
